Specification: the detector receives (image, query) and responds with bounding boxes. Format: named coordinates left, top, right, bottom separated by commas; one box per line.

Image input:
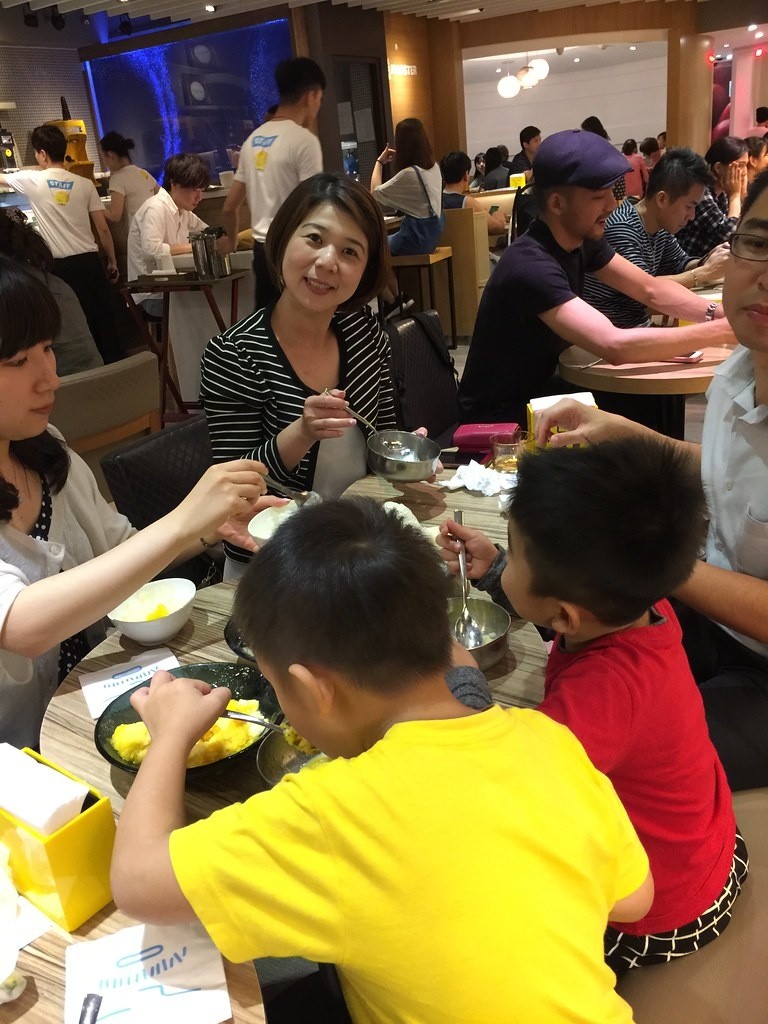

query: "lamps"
left=495, top=57, right=551, bottom=100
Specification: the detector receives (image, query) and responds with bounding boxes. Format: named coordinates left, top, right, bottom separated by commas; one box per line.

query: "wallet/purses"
left=454, top=423, right=521, bottom=454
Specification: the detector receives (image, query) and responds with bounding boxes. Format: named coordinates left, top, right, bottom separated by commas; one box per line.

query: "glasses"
left=727, top=232, right=768, bottom=263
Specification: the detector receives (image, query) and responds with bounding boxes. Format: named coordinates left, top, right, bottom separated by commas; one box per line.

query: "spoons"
left=447, top=507, right=483, bottom=650
left=323, top=388, right=407, bottom=452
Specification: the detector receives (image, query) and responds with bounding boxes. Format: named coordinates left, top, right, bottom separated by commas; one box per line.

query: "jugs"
left=190, top=233, right=220, bottom=282
left=200, top=224, right=232, bottom=277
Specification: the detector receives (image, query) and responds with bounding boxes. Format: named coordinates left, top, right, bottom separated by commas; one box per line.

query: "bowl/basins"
left=247, top=496, right=322, bottom=549
left=443, top=596, right=513, bottom=673
left=93, top=661, right=286, bottom=776
left=256, top=718, right=332, bottom=789
left=106, top=577, right=196, bottom=646
left=365, top=430, right=441, bottom=480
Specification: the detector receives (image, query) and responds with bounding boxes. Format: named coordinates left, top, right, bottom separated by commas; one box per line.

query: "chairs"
left=56, top=352, right=157, bottom=512
left=106, top=411, right=230, bottom=571
left=357, top=311, right=468, bottom=447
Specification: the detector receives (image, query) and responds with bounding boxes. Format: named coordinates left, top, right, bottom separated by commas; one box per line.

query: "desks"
left=1, top=149, right=768, bottom=1024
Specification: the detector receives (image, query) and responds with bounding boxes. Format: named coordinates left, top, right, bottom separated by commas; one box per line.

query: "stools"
left=399, top=242, right=453, bottom=357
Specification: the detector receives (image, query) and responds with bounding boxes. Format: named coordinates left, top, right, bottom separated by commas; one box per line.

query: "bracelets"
left=688, top=269, right=698, bottom=288
left=704, top=302, right=718, bottom=322
left=199, top=536, right=221, bottom=548
left=376, top=160, right=384, bottom=166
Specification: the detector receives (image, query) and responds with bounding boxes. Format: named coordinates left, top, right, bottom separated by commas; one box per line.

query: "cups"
left=489, top=430, right=536, bottom=473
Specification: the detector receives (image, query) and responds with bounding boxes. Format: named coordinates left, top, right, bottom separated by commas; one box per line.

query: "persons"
left=583, top=146, right=731, bottom=330
left=581, top=116, right=667, bottom=199
left=440, top=125, right=542, bottom=274
left=198, top=170, right=443, bottom=583
left=216, top=56, right=326, bottom=312
left=674, top=106, right=768, bottom=257
left=99, top=131, right=161, bottom=231
left=0, top=125, right=129, bottom=365
left=532, top=164, right=767, bottom=795
left=370, top=117, right=447, bottom=256
left=0, top=255, right=292, bottom=753
left=452, top=129, right=740, bottom=425
left=107, top=494, right=654, bottom=1024
left=127, top=153, right=218, bottom=316
left=435, top=434, right=749, bottom=972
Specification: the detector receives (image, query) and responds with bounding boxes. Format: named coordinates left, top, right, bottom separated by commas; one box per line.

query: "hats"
left=533, top=129, right=635, bottom=190
